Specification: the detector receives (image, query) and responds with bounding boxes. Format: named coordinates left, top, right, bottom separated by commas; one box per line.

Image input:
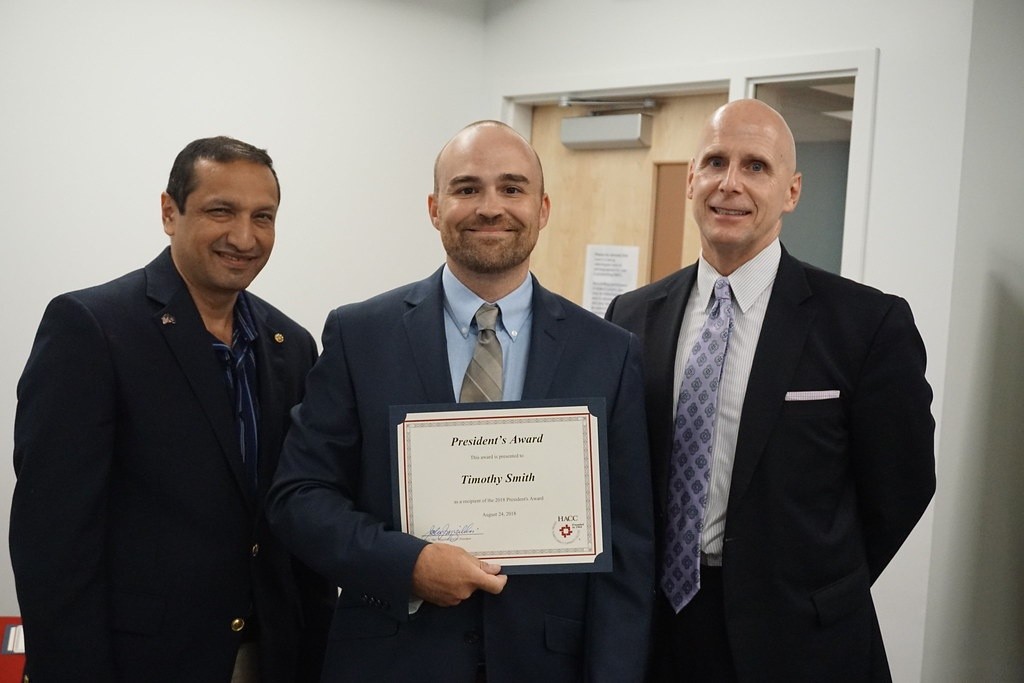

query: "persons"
left=604, top=98, right=936, bottom=683
left=9, top=137, right=319, bottom=683
left=266, top=122, right=656, bottom=683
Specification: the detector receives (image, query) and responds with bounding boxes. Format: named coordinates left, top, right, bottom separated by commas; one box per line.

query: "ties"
left=655, top=276, right=733, bottom=613
left=460, top=302, right=506, bottom=408
